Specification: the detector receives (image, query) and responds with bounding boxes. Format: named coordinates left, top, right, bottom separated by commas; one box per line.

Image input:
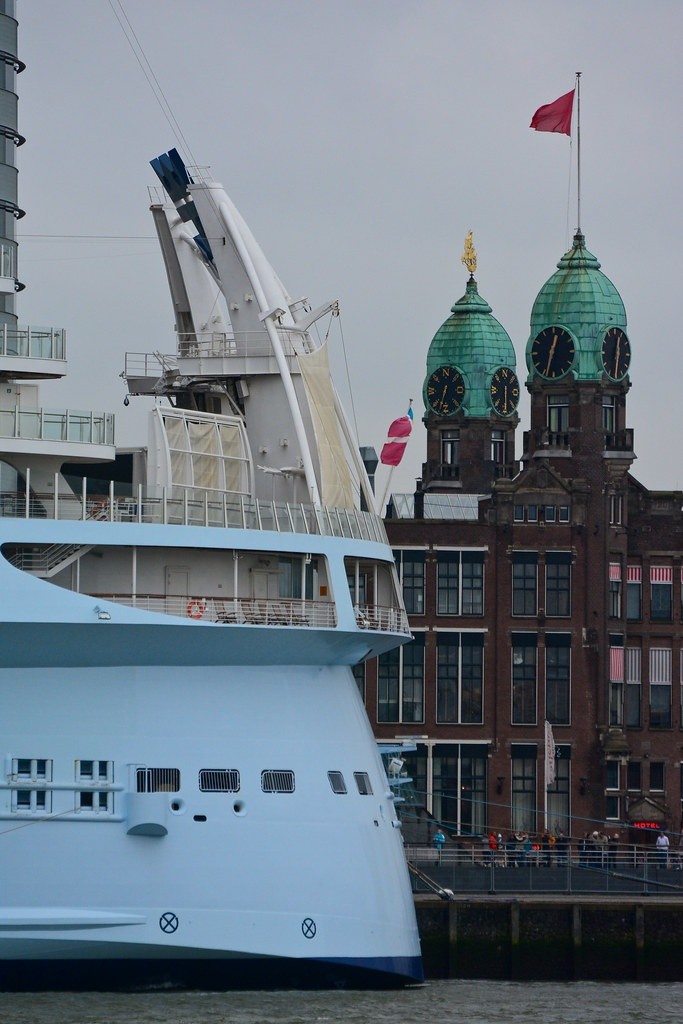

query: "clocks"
left=487, top=365, right=520, bottom=417
left=424, top=365, right=471, bottom=419
left=528, top=326, right=580, bottom=383
left=595, top=326, right=631, bottom=383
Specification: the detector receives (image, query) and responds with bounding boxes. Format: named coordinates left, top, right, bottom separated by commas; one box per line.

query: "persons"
left=655, top=830, right=670, bottom=869
left=553, top=831, right=569, bottom=867
left=482, top=829, right=552, bottom=867
left=576, top=829, right=619, bottom=869
left=431, top=827, right=445, bottom=866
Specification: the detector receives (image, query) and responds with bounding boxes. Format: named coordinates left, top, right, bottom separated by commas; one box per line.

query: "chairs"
left=272, top=604, right=291, bottom=626
left=212, top=601, right=237, bottom=624
left=257, top=602, right=277, bottom=625
left=239, top=600, right=264, bottom=624
left=285, top=603, right=309, bottom=627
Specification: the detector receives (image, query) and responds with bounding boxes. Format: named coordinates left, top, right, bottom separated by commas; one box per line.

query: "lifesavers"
left=187, top=602, right=204, bottom=618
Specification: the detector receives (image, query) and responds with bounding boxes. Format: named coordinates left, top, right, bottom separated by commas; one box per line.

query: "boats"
left=0, top=173, right=433, bottom=991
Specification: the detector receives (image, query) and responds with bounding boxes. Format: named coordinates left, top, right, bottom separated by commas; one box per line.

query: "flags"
left=529, top=89, right=574, bottom=137
